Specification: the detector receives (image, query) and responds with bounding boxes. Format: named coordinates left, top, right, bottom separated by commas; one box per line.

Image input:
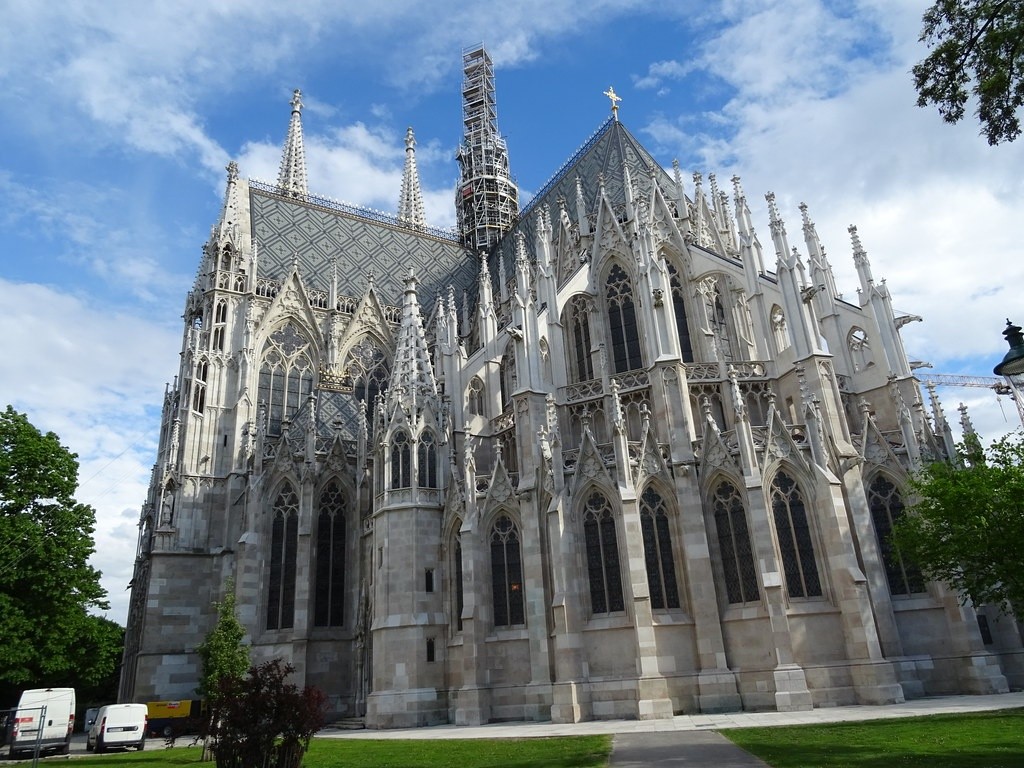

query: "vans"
left=84, top=708, right=101, bottom=733
left=8, top=687, right=76, bottom=759
left=87, top=703, right=149, bottom=753
